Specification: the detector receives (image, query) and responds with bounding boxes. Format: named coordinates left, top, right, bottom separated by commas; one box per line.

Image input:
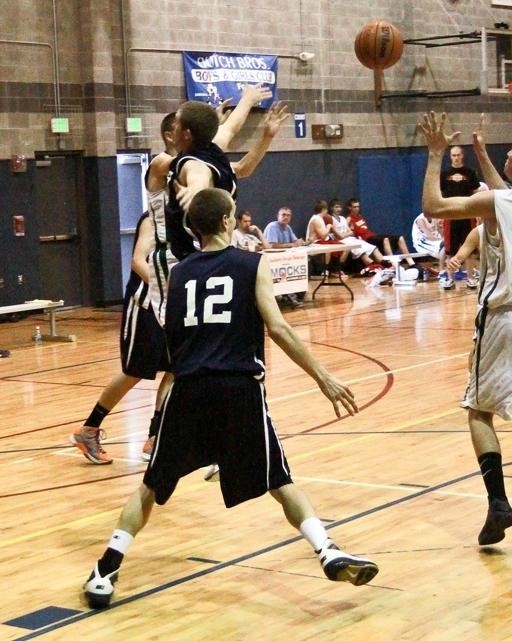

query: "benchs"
left=381, top=253, right=432, bottom=285
left=0, top=299, right=75, bottom=358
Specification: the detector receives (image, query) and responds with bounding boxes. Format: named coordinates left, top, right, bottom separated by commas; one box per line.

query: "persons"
left=418, top=107, right=511, bottom=544
left=71, top=82, right=289, bottom=481
left=82, top=187, right=379, bottom=609
left=231, top=197, right=483, bottom=284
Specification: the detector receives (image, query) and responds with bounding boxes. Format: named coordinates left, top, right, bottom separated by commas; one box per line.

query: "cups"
left=248, top=240, right=255, bottom=252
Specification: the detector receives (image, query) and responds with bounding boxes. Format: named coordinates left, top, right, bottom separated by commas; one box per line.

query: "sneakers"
left=443, top=278, right=457, bottom=291
left=203, top=462, right=222, bottom=485
left=313, top=535, right=380, bottom=587
left=68, top=425, right=114, bottom=465
left=138, top=434, right=157, bottom=462
left=320, top=259, right=428, bottom=283
left=477, top=495, right=512, bottom=547
left=80, top=558, right=122, bottom=602
left=466, top=278, right=477, bottom=288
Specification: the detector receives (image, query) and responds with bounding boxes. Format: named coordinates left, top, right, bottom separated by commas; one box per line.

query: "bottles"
left=423, top=268, right=430, bottom=282
left=33, top=326, right=43, bottom=347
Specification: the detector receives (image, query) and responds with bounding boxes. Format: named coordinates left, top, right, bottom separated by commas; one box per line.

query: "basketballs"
left=356, top=20, right=404, bottom=70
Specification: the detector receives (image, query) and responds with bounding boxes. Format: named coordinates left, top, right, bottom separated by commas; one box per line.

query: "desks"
left=256, top=242, right=361, bottom=301
